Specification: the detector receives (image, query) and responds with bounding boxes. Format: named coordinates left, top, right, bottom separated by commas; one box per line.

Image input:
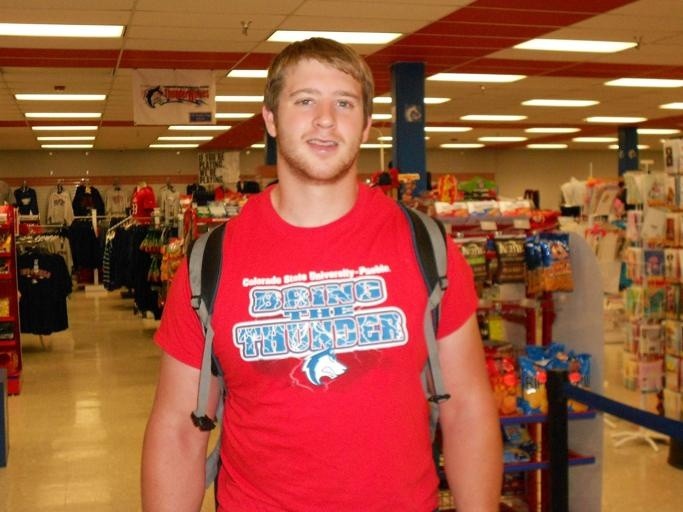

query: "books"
left=621, top=138, right=681, bottom=422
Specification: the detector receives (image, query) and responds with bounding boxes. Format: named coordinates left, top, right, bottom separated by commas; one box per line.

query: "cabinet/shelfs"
left=559, top=158, right=683, bottom=453
left=0, top=204, right=23, bottom=396
left=150, top=207, right=239, bottom=244
left=408, top=197, right=603, bottom=512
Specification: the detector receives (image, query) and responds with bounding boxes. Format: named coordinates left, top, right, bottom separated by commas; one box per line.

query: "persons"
left=131, top=182, right=156, bottom=225
left=558, top=175, right=586, bottom=218
left=141, top=37, right=505, bottom=512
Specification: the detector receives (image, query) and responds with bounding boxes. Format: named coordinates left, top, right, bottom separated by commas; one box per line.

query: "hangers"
left=16, top=216, right=135, bottom=260
